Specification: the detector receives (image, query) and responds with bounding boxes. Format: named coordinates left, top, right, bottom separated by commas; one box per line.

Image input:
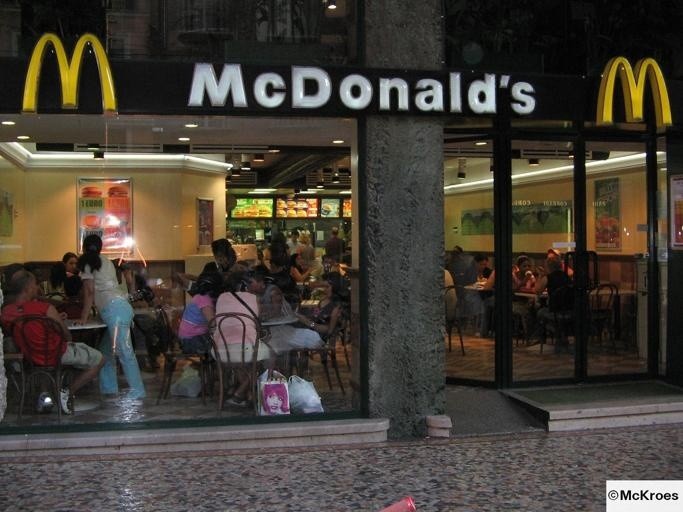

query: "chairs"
left=0, top=262, right=352, bottom=419
left=445, top=277, right=636, bottom=357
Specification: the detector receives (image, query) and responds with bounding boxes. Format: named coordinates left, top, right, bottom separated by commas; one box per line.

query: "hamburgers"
left=234, top=200, right=308, bottom=218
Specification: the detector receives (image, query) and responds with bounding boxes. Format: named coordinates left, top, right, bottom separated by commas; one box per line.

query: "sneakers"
left=97, top=393, right=121, bottom=402
left=59, top=388, right=73, bottom=415
left=226, top=396, right=249, bottom=407
left=36, top=392, right=54, bottom=414
left=115, top=397, right=144, bottom=407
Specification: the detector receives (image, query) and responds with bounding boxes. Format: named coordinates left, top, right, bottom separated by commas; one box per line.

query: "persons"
left=445, top=245, right=575, bottom=347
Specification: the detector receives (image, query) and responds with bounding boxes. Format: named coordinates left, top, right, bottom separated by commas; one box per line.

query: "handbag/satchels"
left=256, top=368, right=291, bottom=414
left=287, top=375, right=325, bottom=413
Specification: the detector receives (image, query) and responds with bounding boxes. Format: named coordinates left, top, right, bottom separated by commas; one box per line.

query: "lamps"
left=291, top=159, right=351, bottom=195
left=454, top=156, right=542, bottom=180
left=224, top=145, right=281, bottom=183
left=82, top=142, right=106, bottom=161
